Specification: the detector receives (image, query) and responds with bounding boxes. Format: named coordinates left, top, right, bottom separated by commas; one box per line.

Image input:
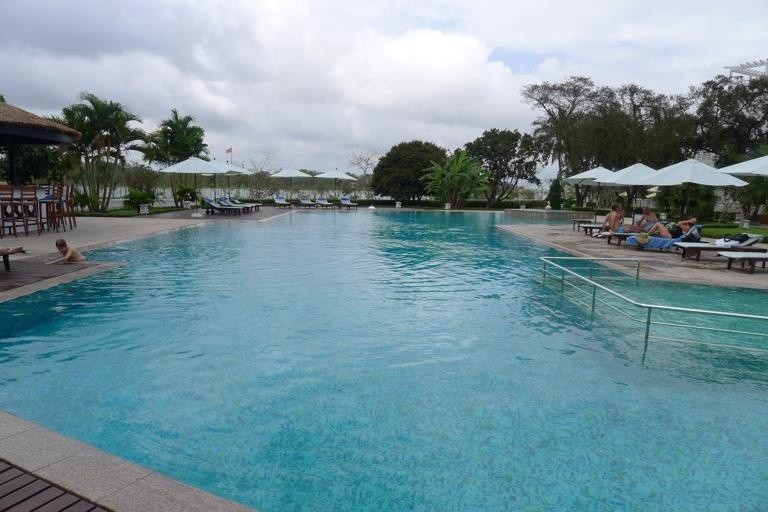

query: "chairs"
left=572, top=214, right=768, bottom=275
left=0, top=182, right=77, bottom=239
left=205, top=195, right=262, bottom=217
left=272, top=196, right=359, bottom=210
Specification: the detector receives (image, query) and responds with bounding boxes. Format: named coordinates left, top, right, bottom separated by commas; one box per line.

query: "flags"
left=226, top=148, right=232, bottom=153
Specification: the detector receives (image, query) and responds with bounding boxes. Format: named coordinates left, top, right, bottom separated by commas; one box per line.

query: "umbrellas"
left=269, top=166, right=360, bottom=197
left=158, top=154, right=256, bottom=202
left=562, top=155, right=768, bottom=226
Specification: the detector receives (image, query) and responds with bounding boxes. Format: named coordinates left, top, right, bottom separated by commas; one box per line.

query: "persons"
left=602, top=202, right=698, bottom=239
left=45, top=238, right=86, bottom=263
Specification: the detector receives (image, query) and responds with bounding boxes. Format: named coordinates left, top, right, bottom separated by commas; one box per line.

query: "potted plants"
left=174, top=183, right=200, bottom=209
left=121, top=185, right=159, bottom=214
